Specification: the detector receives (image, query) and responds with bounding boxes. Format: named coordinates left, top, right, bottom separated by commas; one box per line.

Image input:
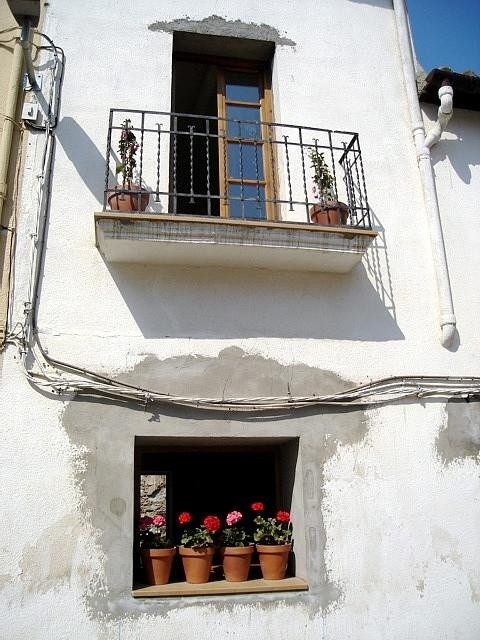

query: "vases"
left=141, top=543, right=292, bottom=586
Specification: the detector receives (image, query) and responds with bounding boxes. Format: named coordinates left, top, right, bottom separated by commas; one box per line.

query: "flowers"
left=138, top=501, right=292, bottom=551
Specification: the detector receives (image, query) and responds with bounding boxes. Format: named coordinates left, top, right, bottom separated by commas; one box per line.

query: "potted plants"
left=304, top=146, right=350, bottom=227
left=108, top=119, right=150, bottom=212
